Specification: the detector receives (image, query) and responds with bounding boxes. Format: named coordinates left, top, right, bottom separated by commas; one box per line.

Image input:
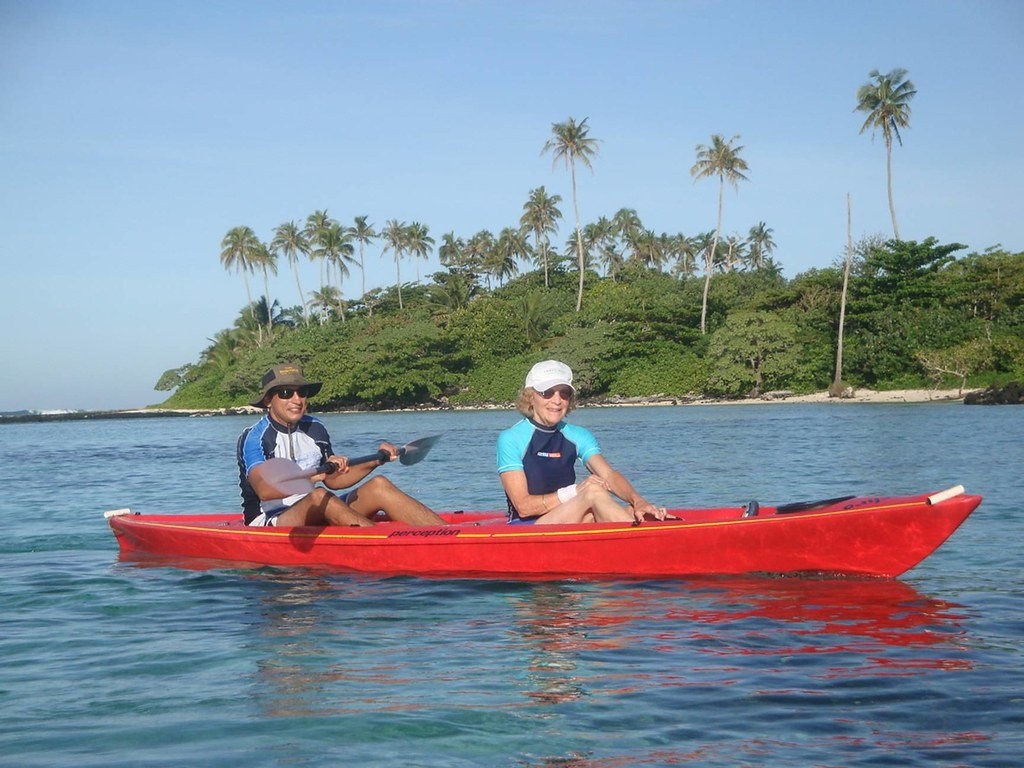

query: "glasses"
left=535, top=387, right=573, bottom=401
left=268, top=388, right=308, bottom=400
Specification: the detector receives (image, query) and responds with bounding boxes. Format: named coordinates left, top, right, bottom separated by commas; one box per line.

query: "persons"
left=237, top=364, right=448, bottom=526
left=496, top=360, right=685, bottom=523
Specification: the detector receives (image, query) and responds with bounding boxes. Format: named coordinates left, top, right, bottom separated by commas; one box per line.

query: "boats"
left=103, top=486, right=983, bottom=583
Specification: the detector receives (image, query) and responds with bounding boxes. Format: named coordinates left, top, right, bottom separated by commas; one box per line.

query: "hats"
left=525, top=360, right=576, bottom=394
left=248, top=364, right=323, bottom=407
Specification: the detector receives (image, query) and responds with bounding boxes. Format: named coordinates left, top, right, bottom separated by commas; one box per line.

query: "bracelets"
left=542, top=494, right=550, bottom=512
left=557, top=483, right=578, bottom=504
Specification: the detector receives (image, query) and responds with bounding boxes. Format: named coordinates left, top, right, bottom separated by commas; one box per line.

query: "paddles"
left=246, top=432, right=445, bottom=502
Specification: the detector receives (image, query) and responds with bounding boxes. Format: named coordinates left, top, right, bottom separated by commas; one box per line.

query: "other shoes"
left=741, top=501, right=759, bottom=519
